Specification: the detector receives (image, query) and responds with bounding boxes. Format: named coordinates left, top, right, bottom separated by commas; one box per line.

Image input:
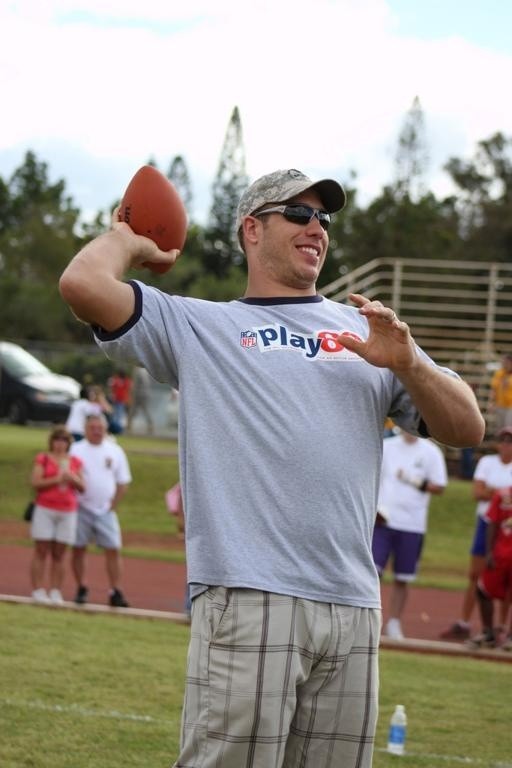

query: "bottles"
left=388, top=702, right=409, bottom=755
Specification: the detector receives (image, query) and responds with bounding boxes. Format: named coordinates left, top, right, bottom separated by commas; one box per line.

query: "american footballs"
left=119, top=165, right=188, bottom=274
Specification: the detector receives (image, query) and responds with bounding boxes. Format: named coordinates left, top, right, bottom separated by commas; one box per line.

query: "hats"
left=235, top=171, right=346, bottom=228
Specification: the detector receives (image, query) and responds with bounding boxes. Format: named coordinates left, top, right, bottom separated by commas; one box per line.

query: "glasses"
left=254, top=202, right=333, bottom=231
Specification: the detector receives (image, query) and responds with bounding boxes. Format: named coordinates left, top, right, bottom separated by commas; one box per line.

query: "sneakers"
left=109, top=587, right=128, bottom=607
left=439, top=622, right=512, bottom=651
left=386, top=617, right=404, bottom=640
left=31, top=587, right=64, bottom=605
left=74, top=586, right=87, bottom=604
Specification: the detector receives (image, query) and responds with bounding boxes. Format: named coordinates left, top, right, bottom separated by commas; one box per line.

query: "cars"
left=1, top=339, right=84, bottom=426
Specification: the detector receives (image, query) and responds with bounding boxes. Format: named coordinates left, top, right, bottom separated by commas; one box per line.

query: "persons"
left=59, top=165, right=487, bottom=768
left=370, top=425, right=512, bottom=652
left=27, top=414, right=130, bottom=607
left=489, top=353, right=512, bottom=429
left=67, top=369, right=131, bottom=444
